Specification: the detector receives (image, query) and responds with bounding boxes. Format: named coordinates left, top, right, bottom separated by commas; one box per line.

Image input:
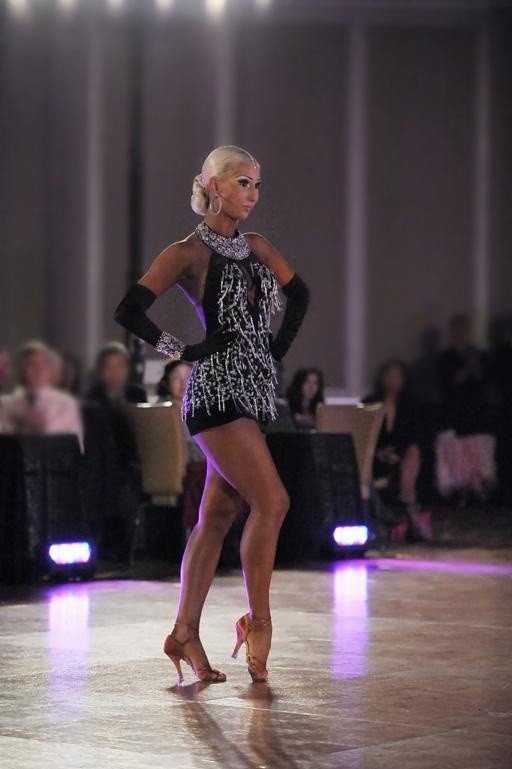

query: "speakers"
left=266, top=431, right=363, bottom=561
left=0, top=433, right=97, bottom=585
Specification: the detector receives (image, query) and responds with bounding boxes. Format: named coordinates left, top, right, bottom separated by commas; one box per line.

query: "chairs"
left=313, top=402, right=384, bottom=562
left=114, top=400, right=199, bottom=575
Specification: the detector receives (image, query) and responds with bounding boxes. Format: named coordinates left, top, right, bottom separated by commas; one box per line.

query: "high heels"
left=163, top=634, right=227, bottom=681
left=232, top=612, right=272, bottom=682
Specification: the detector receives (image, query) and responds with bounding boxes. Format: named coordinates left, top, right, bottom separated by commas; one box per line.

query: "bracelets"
left=154, top=330, right=187, bottom=361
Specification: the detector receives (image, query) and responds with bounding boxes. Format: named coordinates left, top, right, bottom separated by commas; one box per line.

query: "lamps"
left=33, top=533, right=97, bottom=583
left=315, top=525, right=375, bottom=570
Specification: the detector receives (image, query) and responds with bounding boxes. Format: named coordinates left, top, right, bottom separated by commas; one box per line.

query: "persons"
left=288, top=368, right=330, bottom=422
left=113, top=143, right=309, bottom=682
left=0, top=341, right=199, bottom=539
left=359, top=317, right=511, bottom=540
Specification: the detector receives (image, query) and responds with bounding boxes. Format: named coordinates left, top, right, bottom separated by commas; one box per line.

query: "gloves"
left=113, top=283, right=235, bottom=361
